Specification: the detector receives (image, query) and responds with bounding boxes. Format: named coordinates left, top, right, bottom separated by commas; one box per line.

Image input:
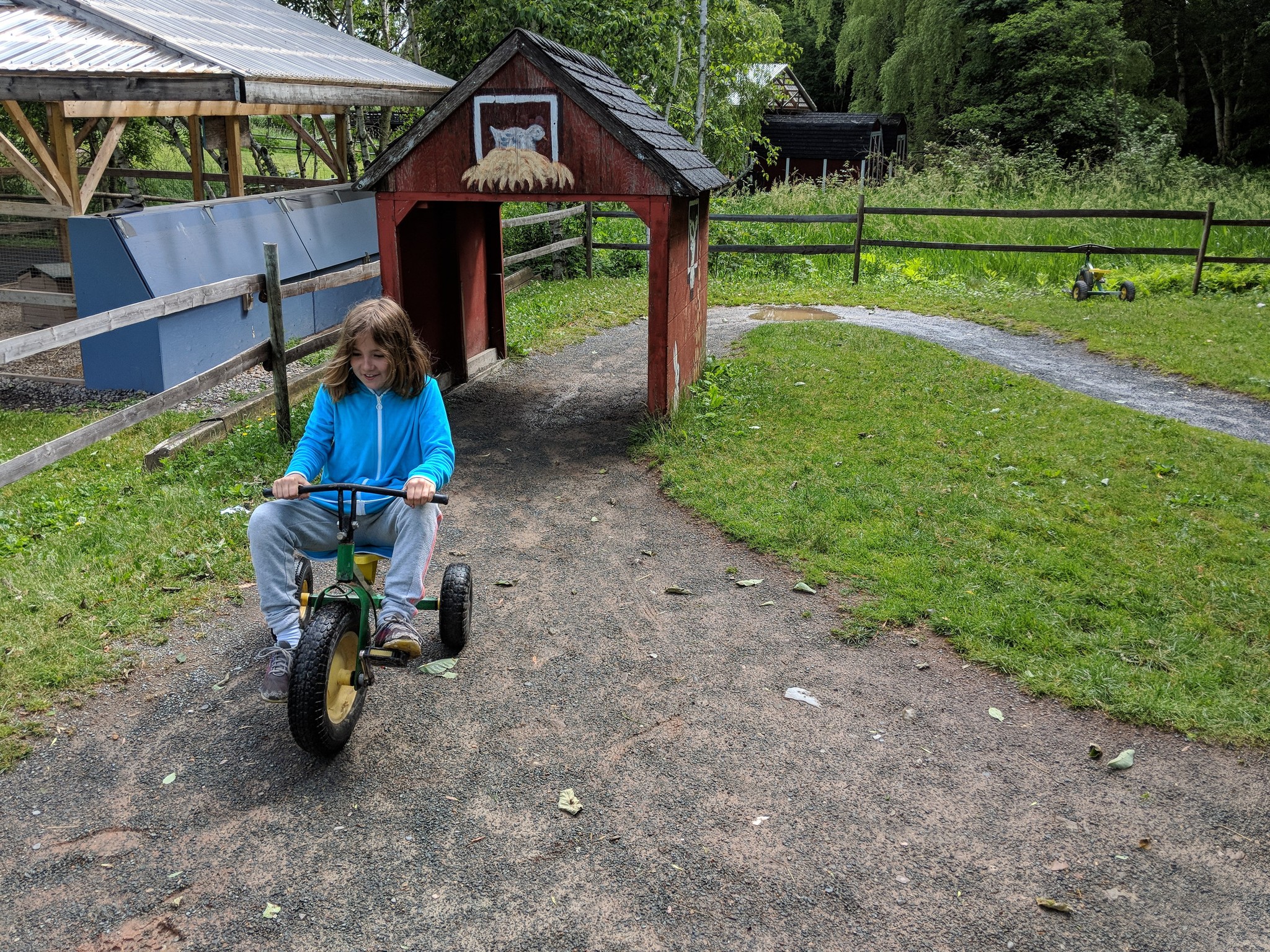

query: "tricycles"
left=1067, top=244, right=1136, bottom=303
left=258, top=481, right=474, bottom=759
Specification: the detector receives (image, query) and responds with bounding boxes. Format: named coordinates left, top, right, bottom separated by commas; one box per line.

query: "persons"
left=247, top=297, right=455, bottom=703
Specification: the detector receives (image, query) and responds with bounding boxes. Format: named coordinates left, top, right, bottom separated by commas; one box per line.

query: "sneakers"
left=258, top=630, right=304, bottom=703
left=371, top=616, right=424, bottom=662
